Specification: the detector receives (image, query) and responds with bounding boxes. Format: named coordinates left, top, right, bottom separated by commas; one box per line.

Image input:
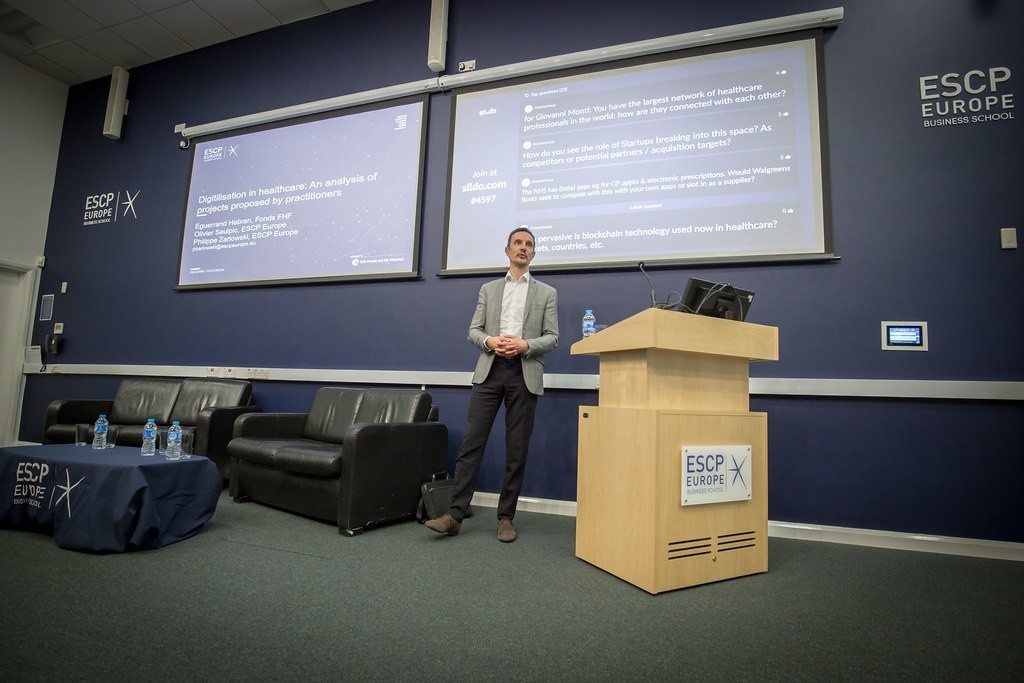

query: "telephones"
left=45, top=334, right=63, bottom=355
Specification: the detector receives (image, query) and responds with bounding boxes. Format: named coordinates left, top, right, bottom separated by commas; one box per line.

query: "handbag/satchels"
left=421, top=471, right=473, bottom=519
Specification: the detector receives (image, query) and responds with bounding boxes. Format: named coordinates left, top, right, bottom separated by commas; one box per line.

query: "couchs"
left=226, top=387, right=450, bottom=536
left=42, top=377, right=262, bottom=479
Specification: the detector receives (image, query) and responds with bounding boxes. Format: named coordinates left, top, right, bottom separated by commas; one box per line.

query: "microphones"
left=637, top=261, right=656, bottom=307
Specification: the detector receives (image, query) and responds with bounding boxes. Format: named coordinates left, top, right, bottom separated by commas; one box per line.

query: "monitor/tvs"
left=678, top=276, right=755, bottom=322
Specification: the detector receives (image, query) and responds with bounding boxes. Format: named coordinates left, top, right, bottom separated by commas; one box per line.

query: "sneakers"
left=497, top=518, right=516, bottom=541
left=425, top=514, right=463, bottom=535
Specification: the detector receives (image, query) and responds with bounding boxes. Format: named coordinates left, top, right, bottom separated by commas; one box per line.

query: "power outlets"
left=458, top=60, right=476, bottom=72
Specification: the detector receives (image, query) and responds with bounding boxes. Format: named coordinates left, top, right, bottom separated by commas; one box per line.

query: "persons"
left=425, top=228, right=559, bottom=543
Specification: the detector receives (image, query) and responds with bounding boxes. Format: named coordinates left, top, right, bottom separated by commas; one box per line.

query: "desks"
left=0, top=443, right=223, bottom=555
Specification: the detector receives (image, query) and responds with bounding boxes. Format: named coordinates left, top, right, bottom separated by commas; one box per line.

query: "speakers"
left=103, top=66, right=130, bottom=140
left=427, top=0, right=449, bottom=72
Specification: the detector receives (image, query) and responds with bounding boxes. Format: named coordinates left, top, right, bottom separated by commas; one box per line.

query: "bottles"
left=141, top=419, right=157, bottom=455
left=92, top=415, right=108, bottom=449
left=582, top=310, right=595, bottom=338
left=166, top=421, right=183, bottom=460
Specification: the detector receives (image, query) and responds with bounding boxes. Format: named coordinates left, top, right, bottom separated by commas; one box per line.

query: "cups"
left=159, top=431, right=168, bottom=455
left=106, top=427, right=121, bottom=448
left=596, top=325, right=608, bottom=333
left=181, top=429, right=193, bottom=459
left=76, top=424, right=89, bottom=446
